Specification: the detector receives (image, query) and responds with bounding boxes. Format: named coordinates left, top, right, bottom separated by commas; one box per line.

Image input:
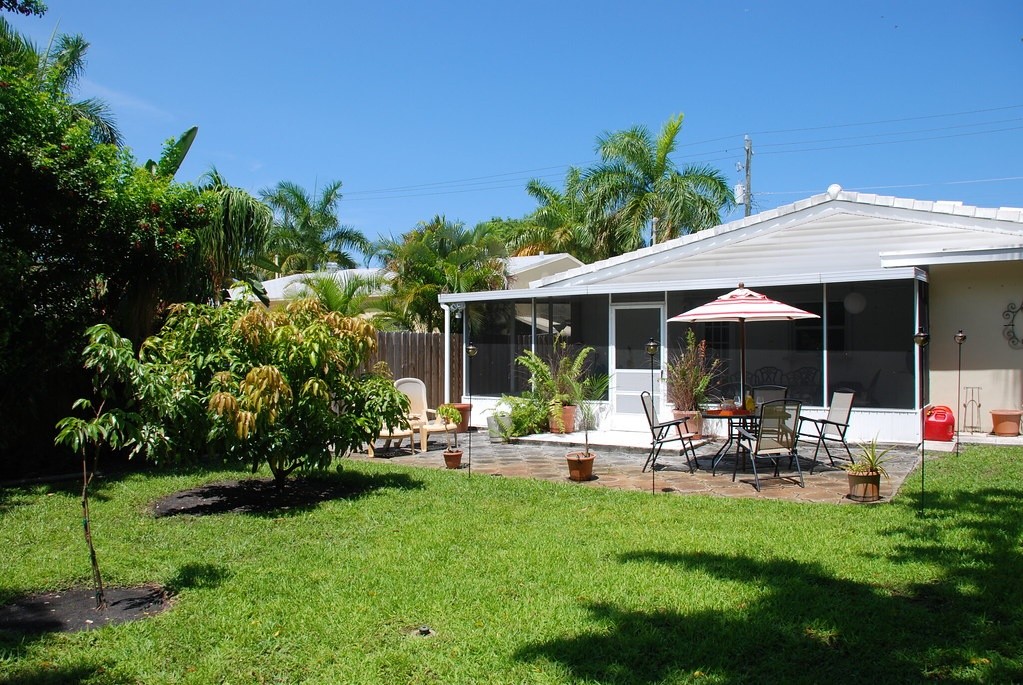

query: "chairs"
left=639, top=391, right=699, bottom=474
left=731, top=366, right=818, bottom=405
left=746, top=385, right=788, bottom=453
left=789, top=390, right=855, bottom=475
left=731, top=399, right=804, bottom=492
left=368, top=419, right=415, bottom=456
left=385, top=377, right=457, bottom=452
left=829, top=369, right=884, bottom=409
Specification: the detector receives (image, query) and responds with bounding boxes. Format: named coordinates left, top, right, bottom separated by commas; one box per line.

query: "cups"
left=734, top=395, right=741, bottom=409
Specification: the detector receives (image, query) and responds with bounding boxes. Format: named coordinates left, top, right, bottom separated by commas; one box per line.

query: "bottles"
left=745, top=391, right=755, bottom=413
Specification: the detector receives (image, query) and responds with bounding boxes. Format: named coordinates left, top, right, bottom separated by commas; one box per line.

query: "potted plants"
left=512, top=333, right=597, bottom=435
left=643, top=328, right=722, bottom=440
left=839, top=432, right=898, bottom=502
left=437, top=402, right=464, bottom=468
left=556, top=376, right=614, bottom=480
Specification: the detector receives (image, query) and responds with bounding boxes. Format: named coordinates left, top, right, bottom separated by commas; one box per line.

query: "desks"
left=700, top=410, right=791, bottom=477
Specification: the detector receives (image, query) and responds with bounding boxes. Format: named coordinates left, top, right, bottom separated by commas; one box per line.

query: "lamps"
left=464, top=342, right=478, bottom=358
left=954, top=328, right=968, bottom=344
left=911, top=326, right=930, bottom=347
left=644, top=337, right=661, bottom=355
left=844, top=286, right=866, bottom=314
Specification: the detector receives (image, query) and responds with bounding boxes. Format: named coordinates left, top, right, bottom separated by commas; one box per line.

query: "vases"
left=990, top=409, right=1023, bottom=437
left=441, top=403, right=472, bottom=433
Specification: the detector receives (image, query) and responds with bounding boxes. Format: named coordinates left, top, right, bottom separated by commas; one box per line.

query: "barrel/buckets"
left=486, top=413, right=512, bottom=442
left=924, top=405, right=955, bottom=441
